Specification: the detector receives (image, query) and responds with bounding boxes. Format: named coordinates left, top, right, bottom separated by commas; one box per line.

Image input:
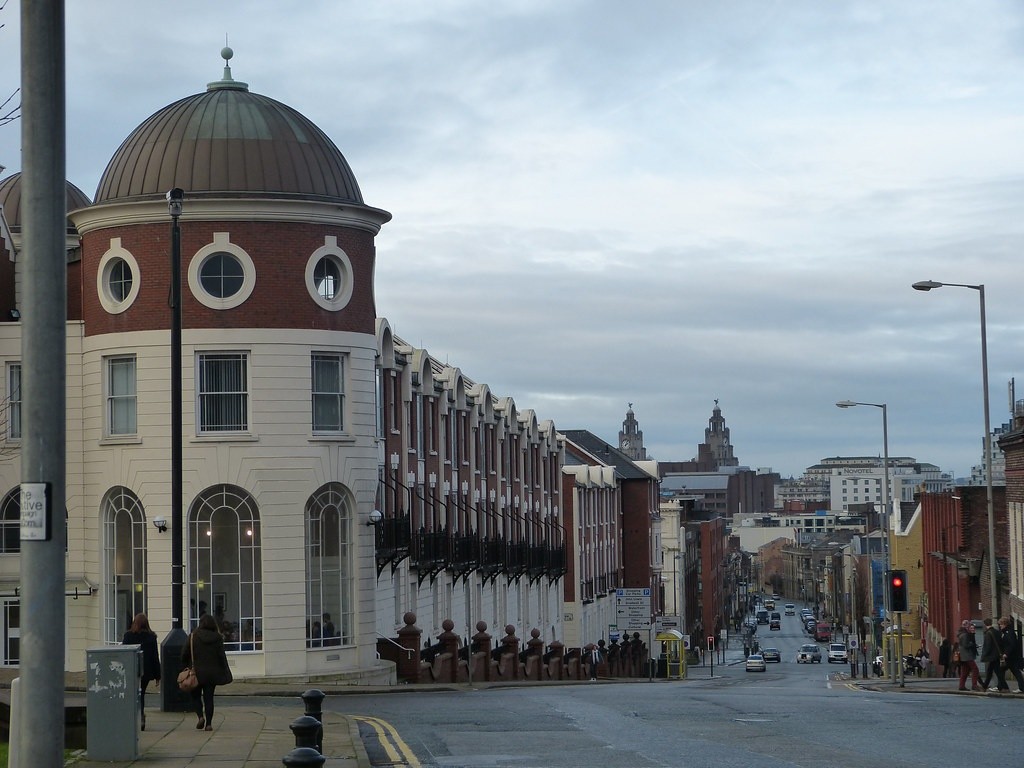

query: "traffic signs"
left=616, top=597, right=652, bottom=631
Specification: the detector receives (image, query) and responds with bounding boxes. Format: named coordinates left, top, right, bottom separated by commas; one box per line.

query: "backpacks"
left=953, top=651, right=960, bottom=662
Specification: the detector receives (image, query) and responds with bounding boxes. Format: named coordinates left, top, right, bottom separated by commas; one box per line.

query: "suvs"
left=874, top=655, right=908, bottom=666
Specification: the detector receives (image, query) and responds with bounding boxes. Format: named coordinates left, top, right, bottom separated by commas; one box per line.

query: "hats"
left=962, top=620, right=971, bottom=628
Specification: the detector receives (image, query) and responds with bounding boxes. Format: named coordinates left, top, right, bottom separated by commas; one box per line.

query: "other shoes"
left=997, top=689, right=1010, bottom=693
left=959, top=687, right=971, bottom=691
left=205, top=725, right=213, bottom=731
left=589, top=678, right=597, bottom=682
left=196, top=717, right=205, bottom=729
left=1012, top=688, right=1023, bottom=693
left=988, top=686, right=999, bottom=692
left=141, top=716, right=146, bottom=731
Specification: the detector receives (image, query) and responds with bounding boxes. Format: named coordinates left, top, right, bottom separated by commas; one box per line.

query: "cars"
left=763, top=648, right=781, bottom=662
left=770, top=612, right=780, bottom=630
left=746, top=655, right=766, bottom=672
left=797, top=645, right=822, bottom=664
left=744, top=622, right=757, bottom=634
left=772, top=594, right=780, bottom=600
left=800, top=609, right=819, bottom=633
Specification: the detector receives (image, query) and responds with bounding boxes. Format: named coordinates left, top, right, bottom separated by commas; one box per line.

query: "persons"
left=123, top=613, right=162, bottom=731
left=735, top=621, right=739, bottom=633
left=957, top=616, right=1024, bottom=694
left=306, top=613, right=345, bottom=647
left=907, top=647, right=931, bottom=677
left=190, top=599, right=263, bottom=651
left=181, top=614, right=233, bottom=731
left=587, top=645, right=605, bottom=681
left=939, top=639, right=961, bottom=678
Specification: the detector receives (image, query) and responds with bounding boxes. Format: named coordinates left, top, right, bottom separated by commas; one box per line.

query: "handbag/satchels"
left=178, top=667, right=199, bottom=690
left=1000, top=657, right=1006, bottom=667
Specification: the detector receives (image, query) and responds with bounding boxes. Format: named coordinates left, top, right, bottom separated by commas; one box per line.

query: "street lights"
left=840, top=516, right=873, bottom=643
left=911, top=280, right=997, bottom=628
left=161, top=188, right=193, bottom=711
left=835, top=400, right=896, bottom=682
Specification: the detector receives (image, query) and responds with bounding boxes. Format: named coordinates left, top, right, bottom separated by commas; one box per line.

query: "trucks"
left=764, top=599, right=775, bottom=610
left=814, top=620, right=831, bottom=641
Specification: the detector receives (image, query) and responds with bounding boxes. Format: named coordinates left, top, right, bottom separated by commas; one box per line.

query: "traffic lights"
left=887, top=569, right=909, bottom=613
left=708, top=637, right=714, bottom=651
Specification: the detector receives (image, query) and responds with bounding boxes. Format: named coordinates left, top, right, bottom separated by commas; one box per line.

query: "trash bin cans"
left=86, top=643, right=142, bottom=761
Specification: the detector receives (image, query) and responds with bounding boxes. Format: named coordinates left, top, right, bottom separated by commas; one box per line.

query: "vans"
left=827, top=643, right=847, bottom=664
left=756, top=611, right=770, bottom=624
left=785, top=604, right=795, bottom=616
